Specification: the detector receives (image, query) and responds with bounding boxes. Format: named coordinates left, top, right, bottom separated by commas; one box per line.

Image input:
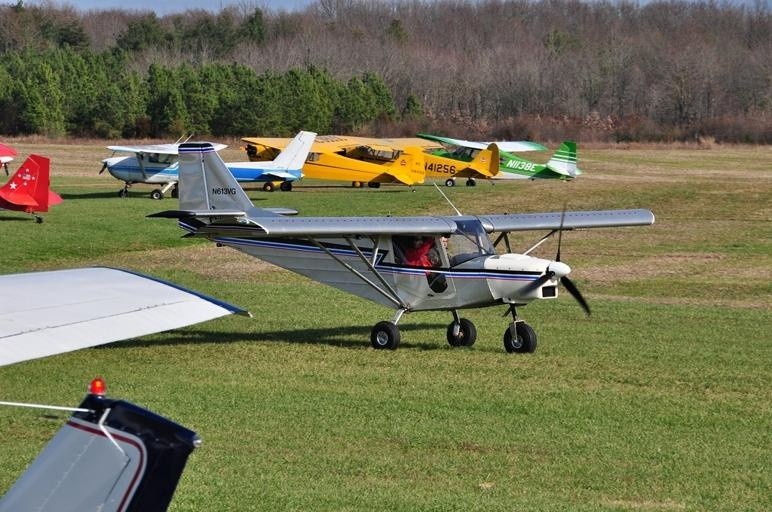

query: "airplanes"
left=0, top=142, right=62, bottom=214
left=147, top=143, right=655, bottom=353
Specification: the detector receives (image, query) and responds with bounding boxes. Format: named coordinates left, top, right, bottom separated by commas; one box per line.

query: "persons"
left=244, top=144, right=257, bottom=157
left=428, top=236, right=454, bottom=267
left=405, top=236, right=447, bottom=293
